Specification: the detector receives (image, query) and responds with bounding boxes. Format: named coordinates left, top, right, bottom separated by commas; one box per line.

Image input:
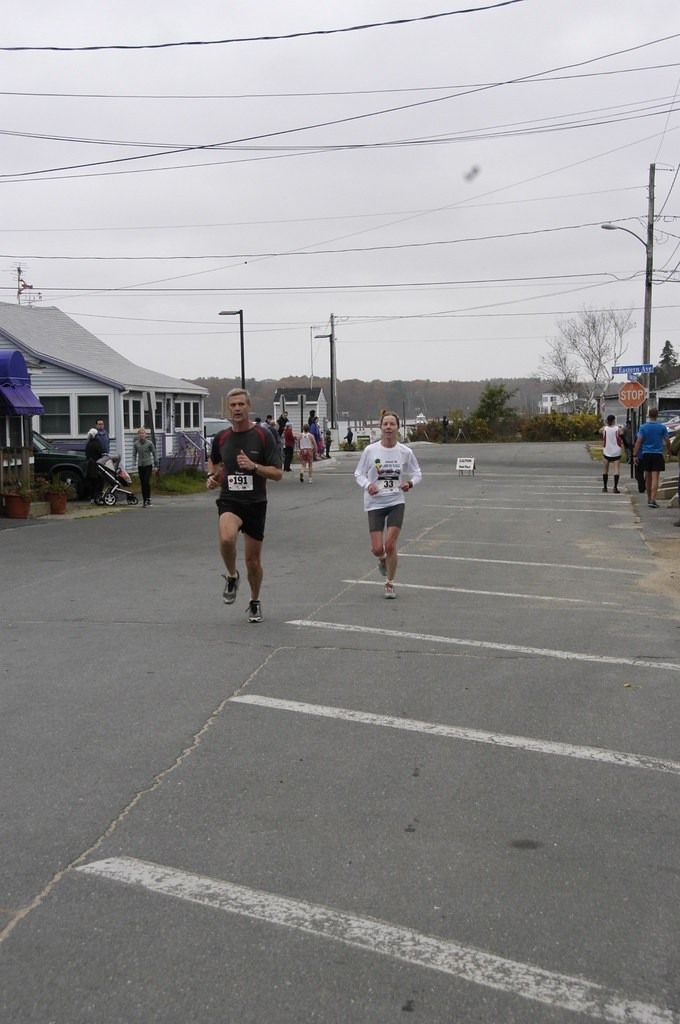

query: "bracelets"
left=252, top=463, right=257, bottom=472
left=633, top=455, right=637, bottom=457
left=207, top=474, right=215, bottom=478
left=408, top=482, right=412, bottom=488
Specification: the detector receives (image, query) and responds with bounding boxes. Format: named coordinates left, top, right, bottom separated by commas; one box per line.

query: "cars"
left=639, top=410, right=680, bottom=439
left=4, top=431, right=122, bottom=502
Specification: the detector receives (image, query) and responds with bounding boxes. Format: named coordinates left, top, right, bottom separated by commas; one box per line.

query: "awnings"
left=0, top=384, right=45, bottom=415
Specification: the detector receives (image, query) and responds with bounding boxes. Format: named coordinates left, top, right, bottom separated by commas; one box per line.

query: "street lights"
left=218, top=309, right=246, bottom=390
left=601, top=223, right=653, bottom=426
left=315, top=334, right=335, bottom=430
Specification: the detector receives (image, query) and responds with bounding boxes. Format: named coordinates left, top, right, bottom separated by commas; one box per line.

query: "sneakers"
left=147, top=498, right=152, bottom=506
left=647, top=501, right=659, bottom=508
left=142, top=501, right=148, bottom=507
left=245, top=599, right=264, bottom=623
left=378, top=557, right=387, bottom=576
left=383, top=581, right=397, bottom=599
left=221, top=569, right=240, bottom=604
left=299, top=472, right=304, bottom=482
left=308, top=479, right=313, bottom=484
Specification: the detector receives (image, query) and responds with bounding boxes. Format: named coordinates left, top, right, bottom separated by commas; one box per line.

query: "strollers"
left=93, top=453, right=139, bottom=506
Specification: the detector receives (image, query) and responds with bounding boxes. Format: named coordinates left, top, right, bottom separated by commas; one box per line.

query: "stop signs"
left=619, top=381, right=646, bottom=409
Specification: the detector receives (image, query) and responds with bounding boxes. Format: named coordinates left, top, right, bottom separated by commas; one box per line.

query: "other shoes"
left=602, top=489, right=608, bottom=492
left=613, top=489, right=620, bottom=493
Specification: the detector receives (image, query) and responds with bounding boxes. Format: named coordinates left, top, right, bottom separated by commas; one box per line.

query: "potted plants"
left=0, top=469, right=40, bottom=519
left=41, top=472, right=74, bottom=515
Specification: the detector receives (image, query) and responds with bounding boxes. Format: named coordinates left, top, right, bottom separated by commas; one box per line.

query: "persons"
left=256, top=411, right=295, bottom=472
left=623, top=421, right=631, bottom=463
left=297, top=424, right=318, bottom=483
left=599, top=415, right=623, bottom=493
left=206, top=388, right=283, bottom=623
left=634, top=432, right=646, bottom=493
left=132, top=429, right=158, bottom=508
left=633, top=408, right=671, bottom=508
left=344, top=427, right=356, bottom=450
left=86, top=419, right=110, bottom=504
left=442, top=416, right=449, bottom=443
left=355, top=409, right=422, bottom=598
left=324, top=430, right=332, bottom=458
left=309, top=410, right=322, bottom=460
